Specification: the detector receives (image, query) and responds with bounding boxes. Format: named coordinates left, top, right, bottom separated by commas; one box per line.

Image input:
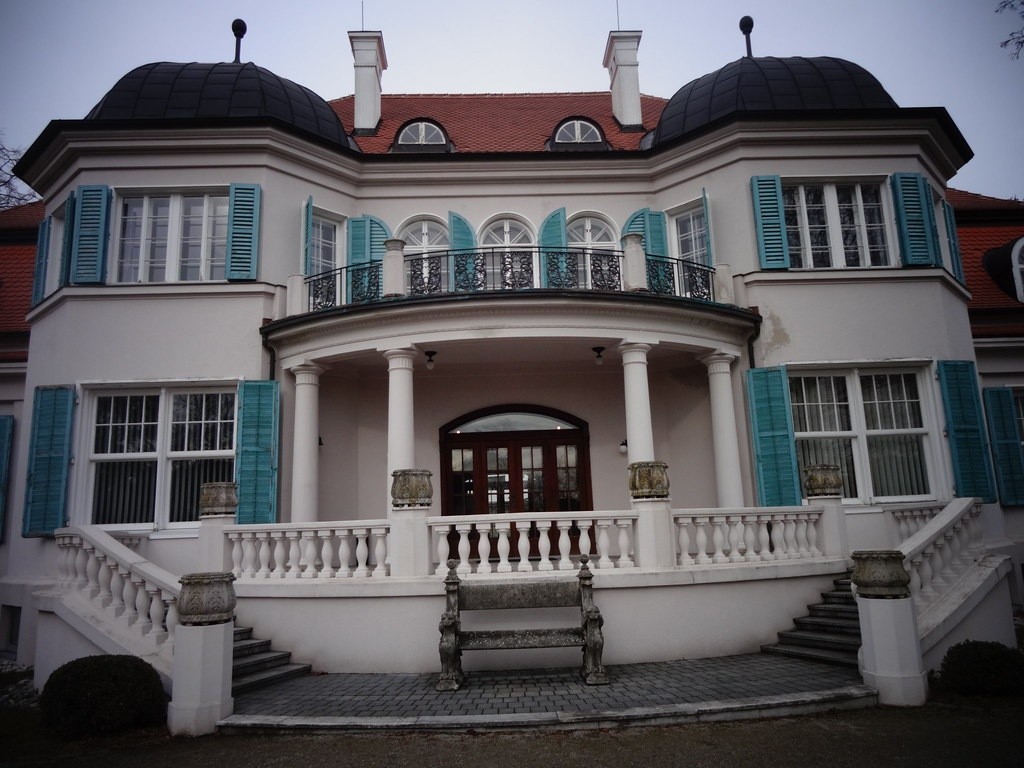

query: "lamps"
left=424, top=351, right=438, bottom=370
left=318, top=436, right=323, bottom=447
left=592, top=346, right=606, bottom=366
left=619, top=439, right=627, bottom=454
